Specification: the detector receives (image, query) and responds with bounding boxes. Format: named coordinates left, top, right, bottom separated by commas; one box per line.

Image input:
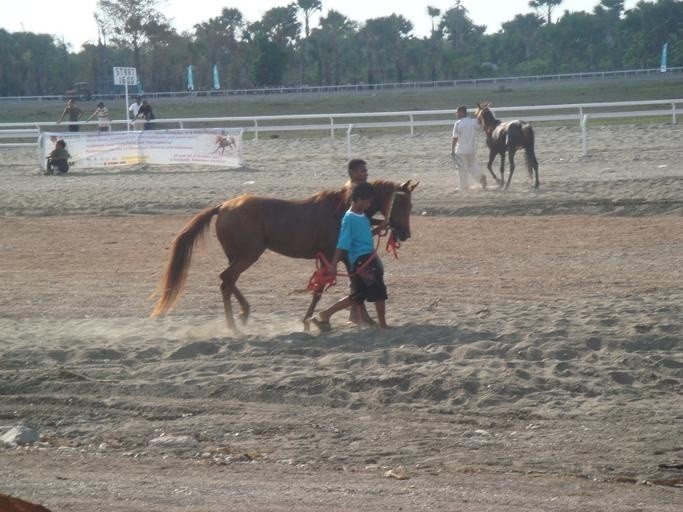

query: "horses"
left=144, top=176, right=420, bottom=337
left=214, top=135, right=237, bottom=153
left=473, top=101, right=542, bottom=190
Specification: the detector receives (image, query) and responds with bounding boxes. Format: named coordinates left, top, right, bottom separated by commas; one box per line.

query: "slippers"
left=310, top=317, right=330, bottom=331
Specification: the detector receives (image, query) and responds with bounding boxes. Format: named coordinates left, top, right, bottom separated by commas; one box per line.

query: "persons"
left=43, top=140, right=69, bottom=176
left=133, top=100, right=155, bottom=130
left=56, top=98, right=82, bottom=132
left=129, top=96, right=145, bottom=130
left=336, top=159, right=376, bottom=326
left=310, top=182, right=388, bottom=331
left=88, top=103, right=110, bottom=131
left=451, top=105, right=487, bottom=191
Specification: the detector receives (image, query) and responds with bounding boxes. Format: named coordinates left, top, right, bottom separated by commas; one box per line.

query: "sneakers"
left=481, top=174, right=487, bottom=190
